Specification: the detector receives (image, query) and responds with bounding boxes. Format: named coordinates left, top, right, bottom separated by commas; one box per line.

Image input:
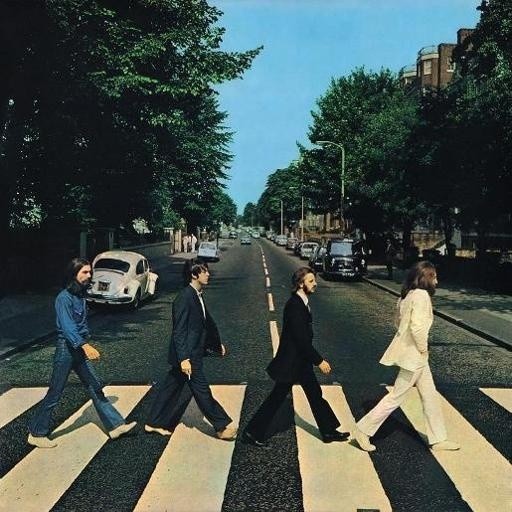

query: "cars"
left=86, top=248, right=164, bottom=311
left=242, top=227, right=367, bottom=280
left=195, top=239, right=220, bottom=262
left=229, top=226, right=241, bottom=240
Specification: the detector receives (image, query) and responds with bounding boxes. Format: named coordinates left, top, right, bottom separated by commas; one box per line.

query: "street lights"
left=315, top=140, right=345, bottom=234
left=269, top=198, right=284, bottom=236
left=288, top=186, right=304, bottom=242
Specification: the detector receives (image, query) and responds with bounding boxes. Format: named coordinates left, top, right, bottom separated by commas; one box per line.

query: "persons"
left=353, top=260, right=461, bottom=452
left=27, top=257, right=137, bottom=449
left=181, top=233, right=197, bottom=254
left=384, top=239, right=396, bottom=281
left=241, top=265, right=350, bottom=447
left=144, top=256, right=238, bottom=440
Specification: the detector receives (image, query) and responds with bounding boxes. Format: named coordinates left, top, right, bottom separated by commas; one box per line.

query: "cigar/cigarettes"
left=188, top=373, right=191, bottom=380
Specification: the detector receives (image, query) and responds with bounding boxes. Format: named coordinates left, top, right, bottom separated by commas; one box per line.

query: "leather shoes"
left=109, top=421, right=137, bottom=440
left=217, top=427, right=238, bottom=439
left=242, top=430, right=269, bottom=446
left=323, top=429, right=350, bottom=442
left=352, top=425, right=377, bottom=451
left=430, top=440, right=461, bottom=451
left=145, top=424, right=172, bottom=435
left=27, top=434, right=59, bottom=449
